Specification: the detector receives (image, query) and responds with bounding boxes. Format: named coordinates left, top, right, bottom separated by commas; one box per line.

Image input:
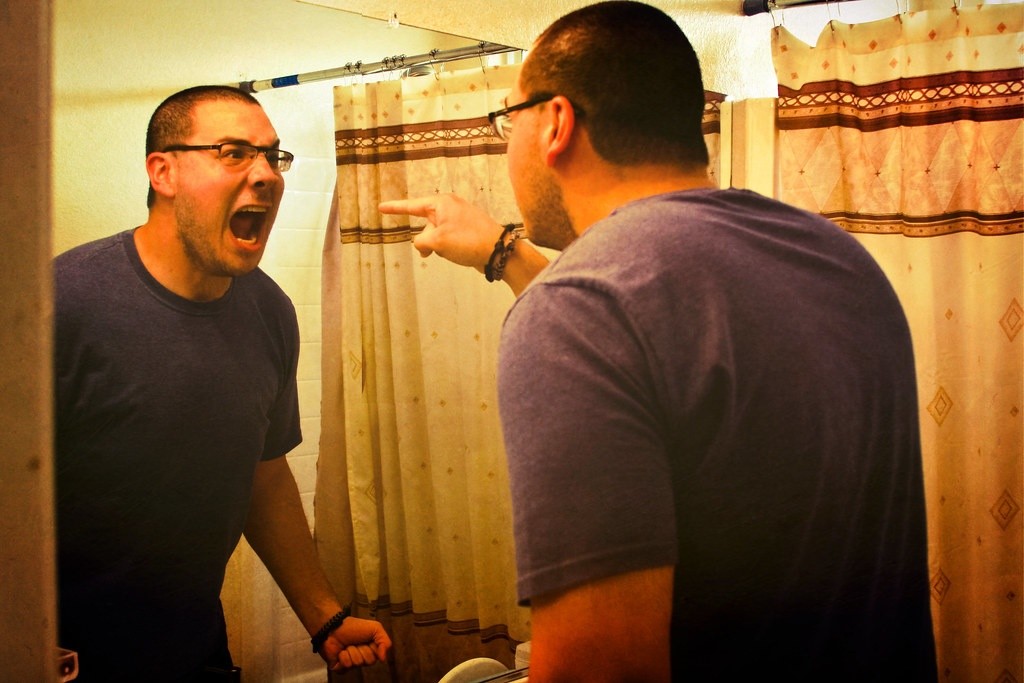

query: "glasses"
left=156, top=140, right=297, bottom=173
left=487, top=93, right=587, bottom=143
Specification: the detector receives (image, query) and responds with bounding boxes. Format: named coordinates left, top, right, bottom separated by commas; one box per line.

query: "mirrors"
left=0, top=0, right=729, bottom=683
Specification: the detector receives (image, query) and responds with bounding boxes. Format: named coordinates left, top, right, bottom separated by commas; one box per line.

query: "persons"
left=51, top=87, right=392, bottom=683
left=375, top=0, right=937, bottom=683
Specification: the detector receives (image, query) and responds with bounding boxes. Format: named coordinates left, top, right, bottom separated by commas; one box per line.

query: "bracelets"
left=480, top=221, right=518, bottom=283
left=496, top=233, right=521, bottom=282
left=310, top=605, right=352, bottom=654
